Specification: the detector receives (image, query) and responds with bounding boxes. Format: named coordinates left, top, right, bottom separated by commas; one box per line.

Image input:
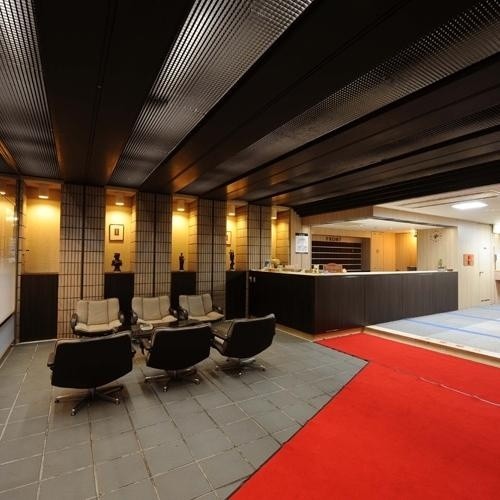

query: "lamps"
left=176, top=199, right=185, bottom=212
left=271, top=207, right=277, bottom=219
left=114, top=192, right=125, bottom=206
left=38, top=183, right=49, bottom=199
left=227, top=204, right=235, bottom=216
left=450, top=200, right=488, bottom=210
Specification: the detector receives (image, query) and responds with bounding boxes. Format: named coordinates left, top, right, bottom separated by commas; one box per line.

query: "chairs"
left=212, top=313, right=276, bottom=377
left=48, top=332, right=136, bottom=417
left=130, top=295, right=179, bottom=342
left=178, top=293, right=227, bottom=327
left=70, top=298, right=130, bottom=337
left=144, top=322, right=214, bottom=393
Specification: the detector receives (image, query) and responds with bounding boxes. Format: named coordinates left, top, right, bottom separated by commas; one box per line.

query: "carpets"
left=231, top=333, right=500, bottom=500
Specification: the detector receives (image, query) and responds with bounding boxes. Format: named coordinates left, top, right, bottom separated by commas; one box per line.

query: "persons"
left=179, top=253, right=184, bottom=270
left=229, top=249, right=235, bottom=269
left=111, top=253, right=122, bottom=272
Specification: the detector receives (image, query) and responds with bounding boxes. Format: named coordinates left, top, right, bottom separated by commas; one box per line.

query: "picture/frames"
left=109, top=223, right=125, bottom=242
left=225, top=231, right=232, bottom=245
